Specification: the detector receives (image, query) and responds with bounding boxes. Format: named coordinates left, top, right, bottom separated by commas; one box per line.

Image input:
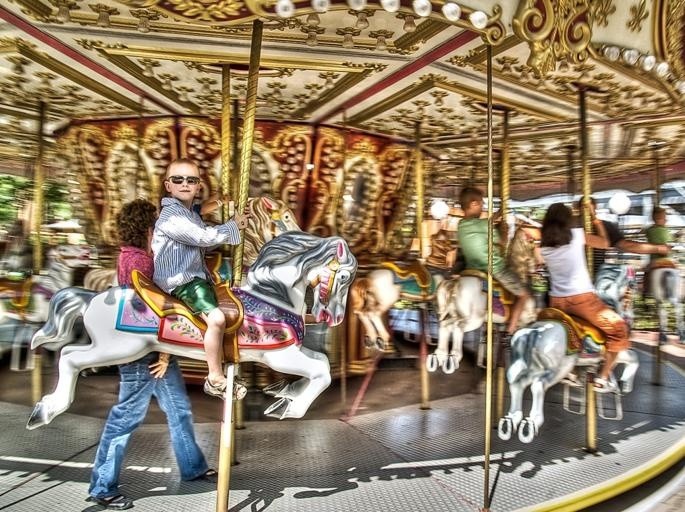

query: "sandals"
left=591, top=377, right=618, bottom=395
left=558, top=371, right=585, bottom=390
left=204, top=374, right=248, bottom=403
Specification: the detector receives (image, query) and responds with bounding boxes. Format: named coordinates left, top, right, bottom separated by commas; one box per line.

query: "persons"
left=534, top=203, right=632, bottom=394
left=638, top=207, right=680, bottom=312
left=87, top=198, right=218, bottom=509
left=572, top=196, right=670, bottom=280
left=150, top=159, right=251, bottom=401
left=193, top=193, right=229, bottom=218
left=457, top=187, right=530, bottom=350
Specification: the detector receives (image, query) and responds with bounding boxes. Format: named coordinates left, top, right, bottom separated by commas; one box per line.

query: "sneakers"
left=200, top=468, right=218, bottom=483
left=93, top=493, right=133, bottom=510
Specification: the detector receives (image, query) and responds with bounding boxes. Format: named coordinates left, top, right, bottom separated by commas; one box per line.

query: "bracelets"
left=214, top=200, right=222, bottom=207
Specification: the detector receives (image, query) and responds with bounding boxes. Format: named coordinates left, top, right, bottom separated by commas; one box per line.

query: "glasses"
left=167, top=174, right=200, bottom=185
